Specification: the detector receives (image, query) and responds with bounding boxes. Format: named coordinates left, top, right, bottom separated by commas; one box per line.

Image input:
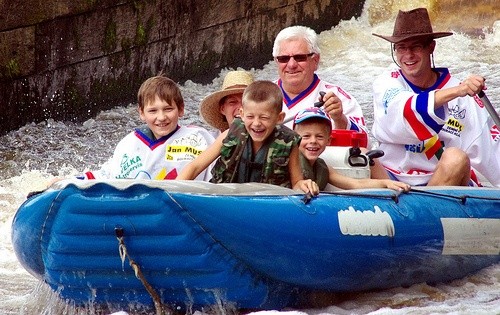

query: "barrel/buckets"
left=318, top=130, right=385, bottom=179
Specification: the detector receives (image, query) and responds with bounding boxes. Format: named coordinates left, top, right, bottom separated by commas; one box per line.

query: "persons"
left=373, top=8, right=500, bottom=188
left=199, top=71, right=257, bottom=133
left=272, top=26, right=372, bottom=149
left=46, top=75, right=207, bottom=187
left=176, top=81, right=319, bottom=196
left=292, top=107, right=411, bottom=193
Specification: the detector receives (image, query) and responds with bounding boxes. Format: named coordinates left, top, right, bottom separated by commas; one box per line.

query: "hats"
left=372, top=7, right=453, bottom=44
left=291, top=107, right=331, bottom=126
left=200, top=71, right=256, bottom=132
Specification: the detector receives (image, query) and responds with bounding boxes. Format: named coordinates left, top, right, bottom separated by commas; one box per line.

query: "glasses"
left=275, top=52, right=314, bottom=63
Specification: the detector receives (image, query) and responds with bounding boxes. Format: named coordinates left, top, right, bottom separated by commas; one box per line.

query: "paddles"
left=280, top=91, right=326, bottom=124
left=478, top=90, right=500, bottom=133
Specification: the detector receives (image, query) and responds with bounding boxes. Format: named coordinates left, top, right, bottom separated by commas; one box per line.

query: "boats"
left=9, top=170, right=496, bottom=315
